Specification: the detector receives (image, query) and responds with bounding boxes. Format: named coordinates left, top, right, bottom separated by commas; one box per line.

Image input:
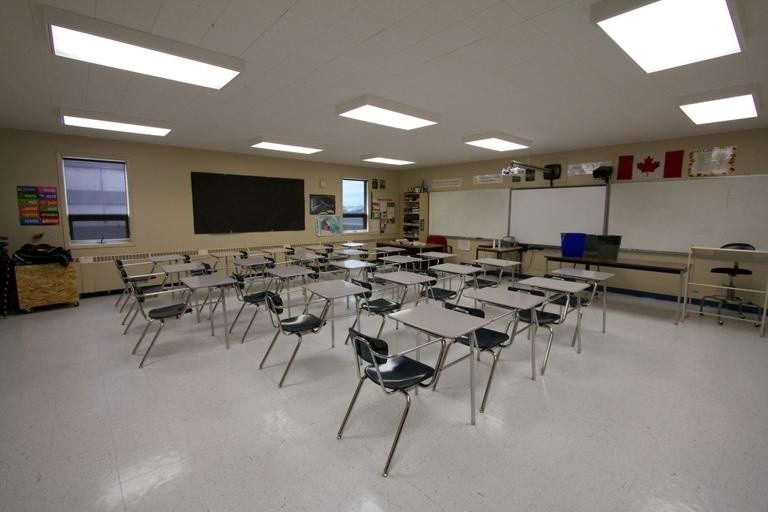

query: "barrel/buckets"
left=423, top=184, right=429, bottom=192
left=561, top=232, right=586, bottom=257
left=597, top=235, right=622, bottom=260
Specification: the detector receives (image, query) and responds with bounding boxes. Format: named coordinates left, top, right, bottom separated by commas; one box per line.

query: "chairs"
left=114, top=235, right=616, bottom=477
left=699, top=243, right=761, bottom=327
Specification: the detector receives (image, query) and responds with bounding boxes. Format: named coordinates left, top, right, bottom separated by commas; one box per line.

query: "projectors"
left=501, top=168, right=525, bottom=176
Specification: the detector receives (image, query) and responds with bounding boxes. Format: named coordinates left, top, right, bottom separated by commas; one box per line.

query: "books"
left=394, top=192, right=425, bottom=246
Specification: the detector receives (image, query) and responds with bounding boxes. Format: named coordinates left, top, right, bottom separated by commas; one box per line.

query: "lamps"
left=39, top=4, right=244, bottom=91
left=57, top=107, right=174, bottom=137
left=463, top=131, right=533, bottom=152
left=672, top=83, right=761, bottom=125
left=335, top=94, right=441, bottom=132
left=249, top=136, right=328, bottom=155
left=590, top=0, right=744, bottom=74
left=361, top=152, right=416, bottom=166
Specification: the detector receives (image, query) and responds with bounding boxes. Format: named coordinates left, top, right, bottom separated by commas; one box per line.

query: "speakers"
left=593, top=166, right=613, bottom=178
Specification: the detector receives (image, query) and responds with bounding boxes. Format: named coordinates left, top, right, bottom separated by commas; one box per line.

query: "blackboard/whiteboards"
left=427, top=188, right=510, bottom=241
left=605, top=174, right=768, bottom=256
left=509, top=184, right=608, bottom=249
left=191, top=171, right=305, bottom=235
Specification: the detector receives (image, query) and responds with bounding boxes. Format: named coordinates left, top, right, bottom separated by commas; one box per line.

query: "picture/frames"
left=310, top=195, right=335, bottom=215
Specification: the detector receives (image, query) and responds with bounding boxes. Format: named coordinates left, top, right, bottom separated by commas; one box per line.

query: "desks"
left=15, top=261, right=81, bottom=310
left=544, top=254, right=692, bottom=324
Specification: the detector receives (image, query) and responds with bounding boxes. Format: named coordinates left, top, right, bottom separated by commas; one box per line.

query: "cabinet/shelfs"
left=400, top=192, right=429, bottom=243
left=681, top=244, right=768, bottom=337
